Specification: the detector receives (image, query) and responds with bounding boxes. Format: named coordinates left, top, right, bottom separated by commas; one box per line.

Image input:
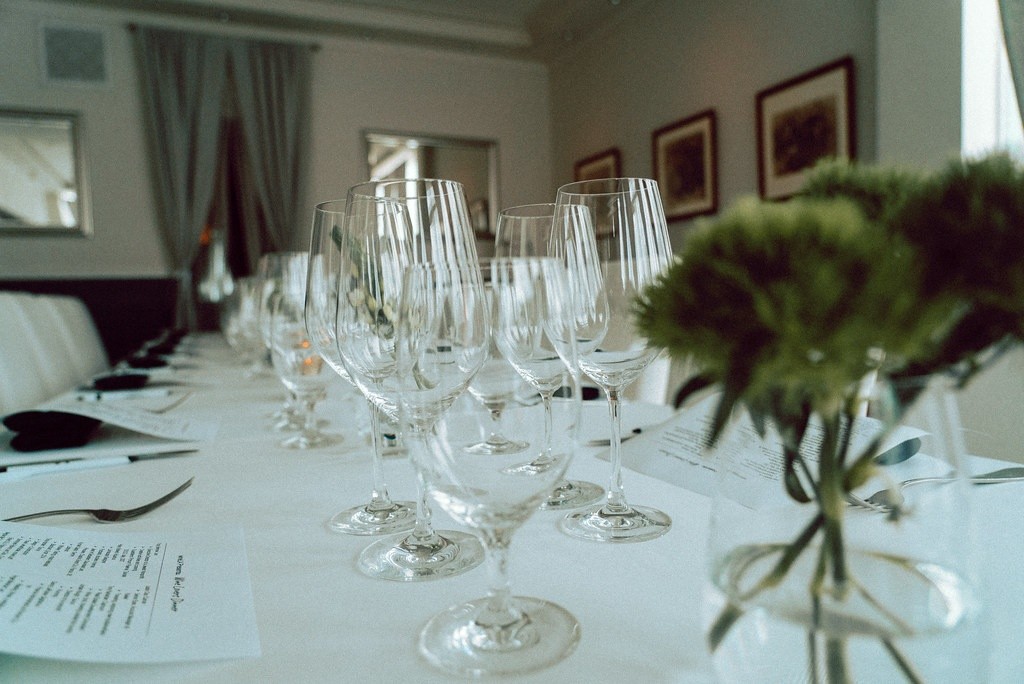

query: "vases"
left=699, top=375, right=989, bottom=684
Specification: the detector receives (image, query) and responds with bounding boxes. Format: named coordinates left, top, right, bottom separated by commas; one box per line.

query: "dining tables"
left=0, top=328, right=1024, bottom=684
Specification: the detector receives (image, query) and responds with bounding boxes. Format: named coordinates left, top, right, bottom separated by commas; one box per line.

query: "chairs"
left=1, top=293, right=48, bottom=421
left=49, top=294, right=111, bottom=383
left=8, top=293, right=80, bottom=395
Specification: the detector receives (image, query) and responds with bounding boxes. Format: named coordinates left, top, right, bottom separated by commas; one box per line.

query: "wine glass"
left=217, top=173, right=681, bottom=679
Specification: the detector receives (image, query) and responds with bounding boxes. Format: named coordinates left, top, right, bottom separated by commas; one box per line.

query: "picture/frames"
left=755, top=56, right=856, bottom=202
left=651, top=108, right=718, bottom=223
left=573, top=146, right=619, bottom=239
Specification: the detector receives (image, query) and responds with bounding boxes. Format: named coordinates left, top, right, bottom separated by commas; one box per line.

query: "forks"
left=83, top=391, right=195, bottom=414
left=2, top=475, right=195, bottom=526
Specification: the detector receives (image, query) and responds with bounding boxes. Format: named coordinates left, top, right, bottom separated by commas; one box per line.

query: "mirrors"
left=1, top=107, right=94, bottom=240
left=364, top=128, right=501, bottom=243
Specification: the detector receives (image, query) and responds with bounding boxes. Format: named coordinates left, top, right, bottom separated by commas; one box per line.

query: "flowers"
left=629, top=145, right=1024, bottom=377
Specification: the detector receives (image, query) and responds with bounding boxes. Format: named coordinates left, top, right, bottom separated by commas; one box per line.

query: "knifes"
left=0, top=449, right=200, bottom=477
